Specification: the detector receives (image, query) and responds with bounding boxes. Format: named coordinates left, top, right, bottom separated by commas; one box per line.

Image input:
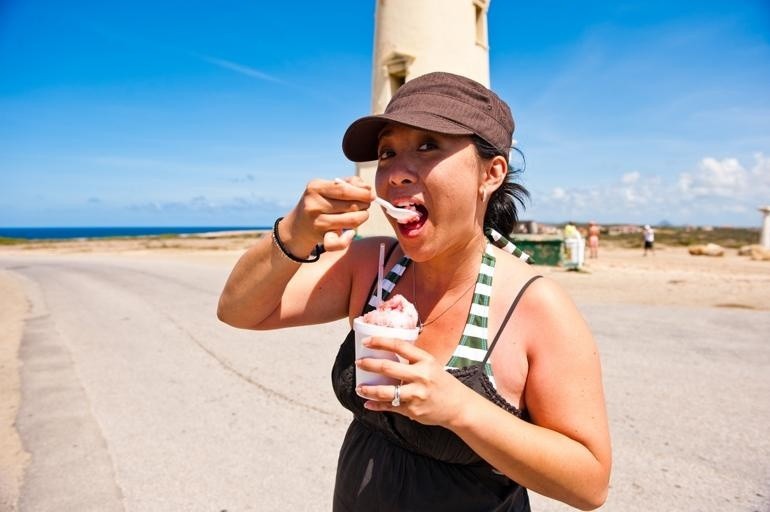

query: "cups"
left=353, top=316, right=422, bottom=402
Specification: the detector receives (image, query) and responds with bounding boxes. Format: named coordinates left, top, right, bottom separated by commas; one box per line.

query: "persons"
left=563, top=218, right=582, bottom=264
left=213, top=68, right=616, bottom=510
left=641, top=224, right=657, bottom=258
left=586, top=219, right=601, bottom=259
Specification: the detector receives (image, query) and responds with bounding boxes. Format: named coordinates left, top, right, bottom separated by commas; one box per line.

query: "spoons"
left=334, top=176, right=426, bottom=224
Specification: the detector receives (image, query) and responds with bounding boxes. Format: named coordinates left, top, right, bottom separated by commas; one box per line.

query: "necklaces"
left=409, top=261, right=480, bottom=342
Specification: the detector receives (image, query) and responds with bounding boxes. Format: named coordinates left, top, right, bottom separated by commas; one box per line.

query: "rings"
left=390, top=382, right=403, bottom=410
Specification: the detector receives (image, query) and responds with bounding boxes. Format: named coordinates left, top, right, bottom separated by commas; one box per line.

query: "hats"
left=343, top=73, right=515, bottom=163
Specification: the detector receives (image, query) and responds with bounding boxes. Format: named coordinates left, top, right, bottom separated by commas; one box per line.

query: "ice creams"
left=362, top=293, right=419, bottom=330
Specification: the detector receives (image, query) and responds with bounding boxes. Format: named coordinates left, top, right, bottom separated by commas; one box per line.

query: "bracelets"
left=270, top=209, right=329, bottom=264
